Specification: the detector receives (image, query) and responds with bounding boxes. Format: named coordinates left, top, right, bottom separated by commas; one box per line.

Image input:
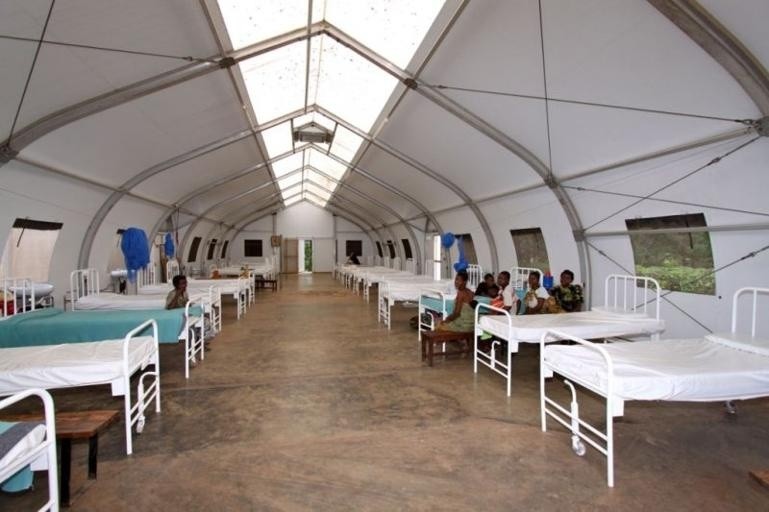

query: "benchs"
left=255, top=280, right=276, bottom=292
left=2, top=412, right=121, bottom=509
left=421, top=327, right=472, bottom=364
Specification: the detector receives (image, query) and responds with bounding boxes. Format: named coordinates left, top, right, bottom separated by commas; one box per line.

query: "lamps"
left=300, top=132, right=328, bottom=143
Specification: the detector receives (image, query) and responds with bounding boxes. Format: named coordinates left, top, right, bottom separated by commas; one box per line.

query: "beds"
left=418, top=268, right=544, bottom=357
left=137, top=258, right=273, bottom=321
left=1, top=277, right=206, bottom=378
left=474, top=274, right=665, bottom=397
left=1, top=386, right=61, bottom=512
left=69, top=268, right=222, bottom=332
left=332, top=255, right=767, bottom=487
left=332, top=256, right=446, bottom=304
left=1, top=318, right=161, bottom=455
left=540, top=288, right=769, bottom=487
left=377, top=264, right=482, bottom=328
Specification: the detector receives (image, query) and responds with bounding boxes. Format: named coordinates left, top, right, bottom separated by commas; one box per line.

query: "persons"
left=428, top=268, right=584, bottom=360
left=165, top=275, right=216, bottom=353
